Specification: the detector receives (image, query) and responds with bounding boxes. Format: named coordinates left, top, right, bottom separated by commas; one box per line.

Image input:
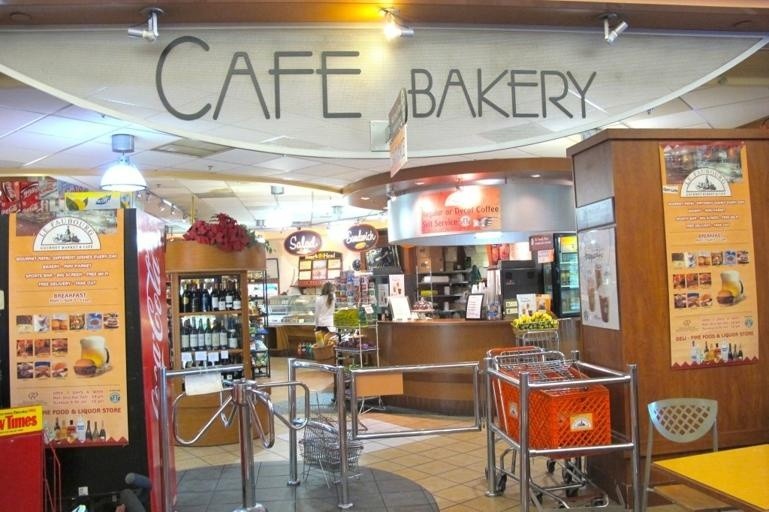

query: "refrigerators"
left=543, top=232, right=581, bottom=316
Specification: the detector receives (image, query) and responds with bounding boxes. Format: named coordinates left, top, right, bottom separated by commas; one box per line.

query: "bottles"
left=44, top=416, right=108, bottom=442
left=179, top=315, right=239, bottom=351
left=179, top=274, right=242, bottom=314
left=689, top=338, right=744, bottom=365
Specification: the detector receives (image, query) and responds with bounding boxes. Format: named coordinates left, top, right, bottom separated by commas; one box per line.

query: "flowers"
left=183, top=213, right=253, bottom=251
left=510, top=312, right=558, bottom=331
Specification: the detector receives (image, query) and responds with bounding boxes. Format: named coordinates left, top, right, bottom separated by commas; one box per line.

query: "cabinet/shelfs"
left=167, top=237, right=271, bottom=446
left=417, top=270, right=471, bottom=315
left=332, top=311, right=386, bottom=417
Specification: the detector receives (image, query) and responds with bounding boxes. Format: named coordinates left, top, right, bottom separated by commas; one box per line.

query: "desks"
left=652, top=444, right=769, bottom=512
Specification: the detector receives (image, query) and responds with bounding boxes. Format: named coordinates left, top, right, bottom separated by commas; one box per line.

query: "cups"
left=597, top=285, right=613, bottom=322
left=586, top=278, right=596, bottom=312
left=594, top=261, right=609, bottom=288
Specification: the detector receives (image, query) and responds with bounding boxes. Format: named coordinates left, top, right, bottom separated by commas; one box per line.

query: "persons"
left=314, top=282, right=335, bottom=331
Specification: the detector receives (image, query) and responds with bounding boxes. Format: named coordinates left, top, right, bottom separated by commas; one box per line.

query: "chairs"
left=644, top=397, right=717, bottom=512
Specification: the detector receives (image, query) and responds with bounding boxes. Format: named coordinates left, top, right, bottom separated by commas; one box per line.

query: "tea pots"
left=79, top=335, right=110, bottom=369
left=720, top=271, right=744, bottom=299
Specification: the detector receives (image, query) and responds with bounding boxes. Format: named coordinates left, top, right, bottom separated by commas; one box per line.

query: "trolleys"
left=486, top=344, right=611, bottom=509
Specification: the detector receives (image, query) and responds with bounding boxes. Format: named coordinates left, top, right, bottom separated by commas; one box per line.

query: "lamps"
left=101, top=133, right=146, bottom=192
